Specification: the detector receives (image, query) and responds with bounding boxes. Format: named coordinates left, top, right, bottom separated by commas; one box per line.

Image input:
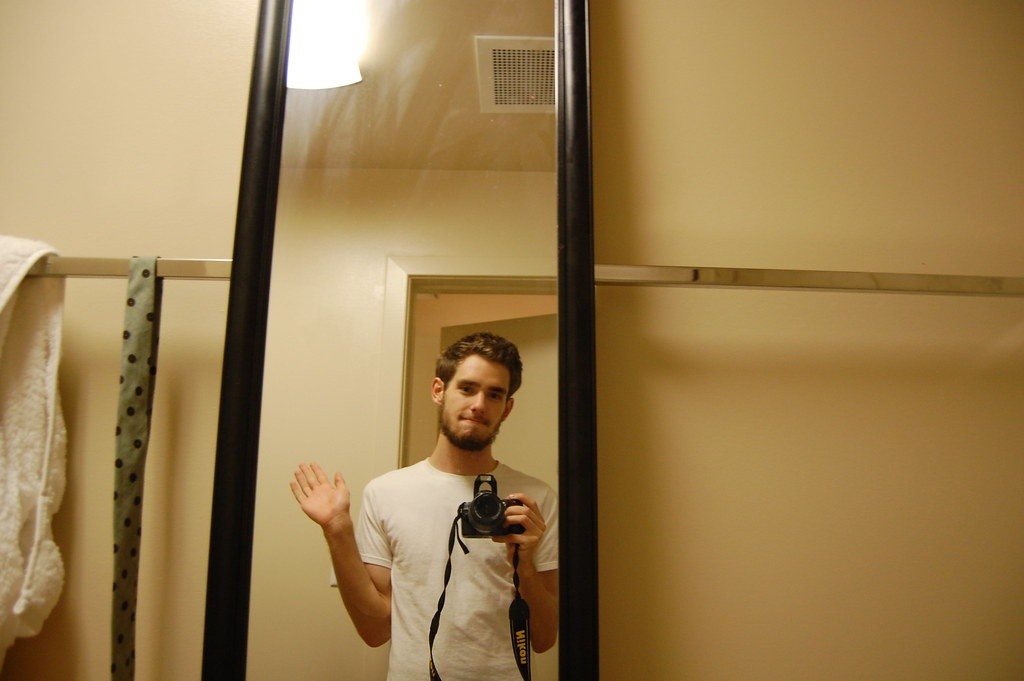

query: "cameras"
left=461, top=474, right=525, bottom=539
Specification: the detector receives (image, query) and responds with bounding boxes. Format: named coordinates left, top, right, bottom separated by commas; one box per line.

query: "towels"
left=0, top=230, right=69, bottom=659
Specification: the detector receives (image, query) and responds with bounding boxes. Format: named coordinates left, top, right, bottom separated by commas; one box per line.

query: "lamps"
left=287, top=0, right=363, bottom=90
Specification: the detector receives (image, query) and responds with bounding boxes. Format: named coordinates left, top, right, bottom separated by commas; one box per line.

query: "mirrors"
left=197, top=0, right=598, bottom=681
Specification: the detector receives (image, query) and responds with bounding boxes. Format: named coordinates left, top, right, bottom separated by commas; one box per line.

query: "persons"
left=289, top=332, right=560, bottom=681
